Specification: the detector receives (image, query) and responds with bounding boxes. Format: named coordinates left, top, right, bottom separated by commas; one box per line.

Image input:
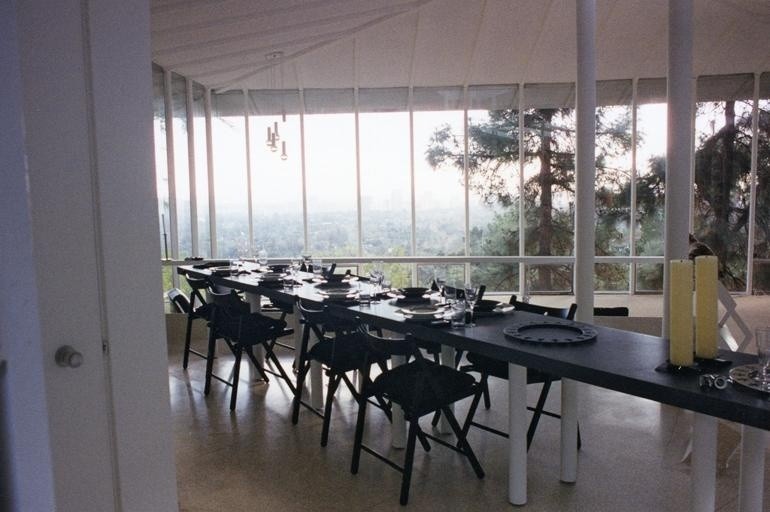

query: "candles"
left=667, top=257, right=721, bottom=371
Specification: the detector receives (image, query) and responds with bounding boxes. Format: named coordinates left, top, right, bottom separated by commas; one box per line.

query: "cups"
left=283, top=277, right=293, bottom=294
left=312, top=258, right=322, bottom=283
left=380, top=271, right=391, bottom=295
left=359, top=281, right=370, bottom=308
left=451, top=304, right=465, bottom=330
left=444, top=288, right=457, bottom=305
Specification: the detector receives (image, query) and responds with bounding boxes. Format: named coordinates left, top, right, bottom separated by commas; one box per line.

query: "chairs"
left=171, top=264, right=578, bottom=506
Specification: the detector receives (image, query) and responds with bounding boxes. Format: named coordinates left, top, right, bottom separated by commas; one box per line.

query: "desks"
left=175, top=260, right=769, bottom=509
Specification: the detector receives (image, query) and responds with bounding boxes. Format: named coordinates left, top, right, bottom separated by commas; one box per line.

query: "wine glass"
left=463, top=286, right=476, bottom=328
left=435, top=275, right=448, bottom=306
left=751, top=325, right=770, bottom=389
left=317, top=289, right=357, bottom=299
left=289, top=264, right=300, bottom=288
left=301, top=249, right=313, bottom=281
left=370, top=270, right=383, bottom=304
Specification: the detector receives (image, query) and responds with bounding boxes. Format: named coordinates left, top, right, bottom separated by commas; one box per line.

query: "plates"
left=473, top=300, right=515, bottom=316
left=400, top=304, right=445, bottom=318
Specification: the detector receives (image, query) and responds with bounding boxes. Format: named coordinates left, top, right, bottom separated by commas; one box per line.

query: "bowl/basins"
left=398, top=288, right=428, bottom=299
left=321, top=274, right=348, bottom=284
